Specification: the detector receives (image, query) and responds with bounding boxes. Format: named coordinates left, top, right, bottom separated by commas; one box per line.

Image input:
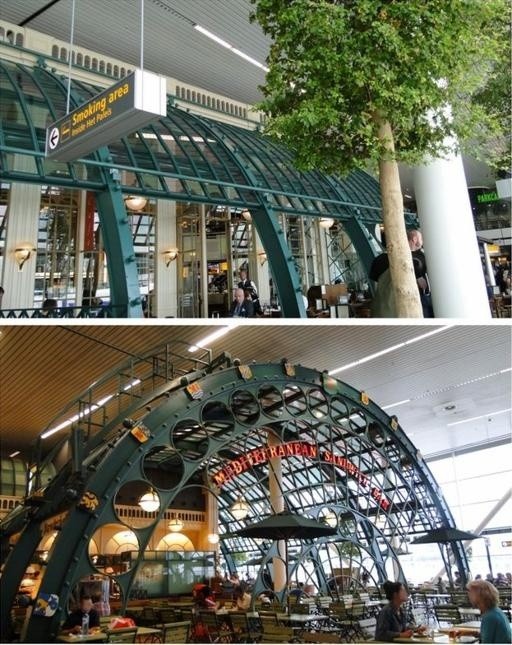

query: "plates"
left=455, top=636, right=479, bottom=643
left=412, top=632, right=429, bottom=637
left=79, top=635, right=93, bottom=638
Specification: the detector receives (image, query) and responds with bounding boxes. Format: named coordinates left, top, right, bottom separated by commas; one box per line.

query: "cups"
left=448, top=631, right=456, bottom=641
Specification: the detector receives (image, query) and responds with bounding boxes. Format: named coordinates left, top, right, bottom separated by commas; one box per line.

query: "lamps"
left=233, top=491, right=249, bottom=521
left=11, top=237, right=35, bottom=269
left=161, top=245, right=181, bottom=268
left=320, top=216, right=337, bottom=229
left=123, top=190, right=153, bottom=212
left=241, top=209, right=253, bottom=222
left=138, top=489, right=164, bottom=514
left=324, top=511, right=338, bottom=533
left=168, top=511, right=186, bottom=533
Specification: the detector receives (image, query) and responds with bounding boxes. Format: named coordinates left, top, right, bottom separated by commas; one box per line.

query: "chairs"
left=14, top=557, right=511, bottom=644
left=54, top=279, right=510, bottom=317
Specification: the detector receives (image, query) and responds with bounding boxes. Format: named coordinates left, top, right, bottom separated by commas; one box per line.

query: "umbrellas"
left=408, top=526, right=485, bottom=584
left=234, top=506, right=338, bottom=615
left=241, top=555, right=298, bottom=576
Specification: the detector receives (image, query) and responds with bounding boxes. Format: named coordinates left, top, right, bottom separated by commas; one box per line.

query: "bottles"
left=82, top=614, right=89, bottom=639
